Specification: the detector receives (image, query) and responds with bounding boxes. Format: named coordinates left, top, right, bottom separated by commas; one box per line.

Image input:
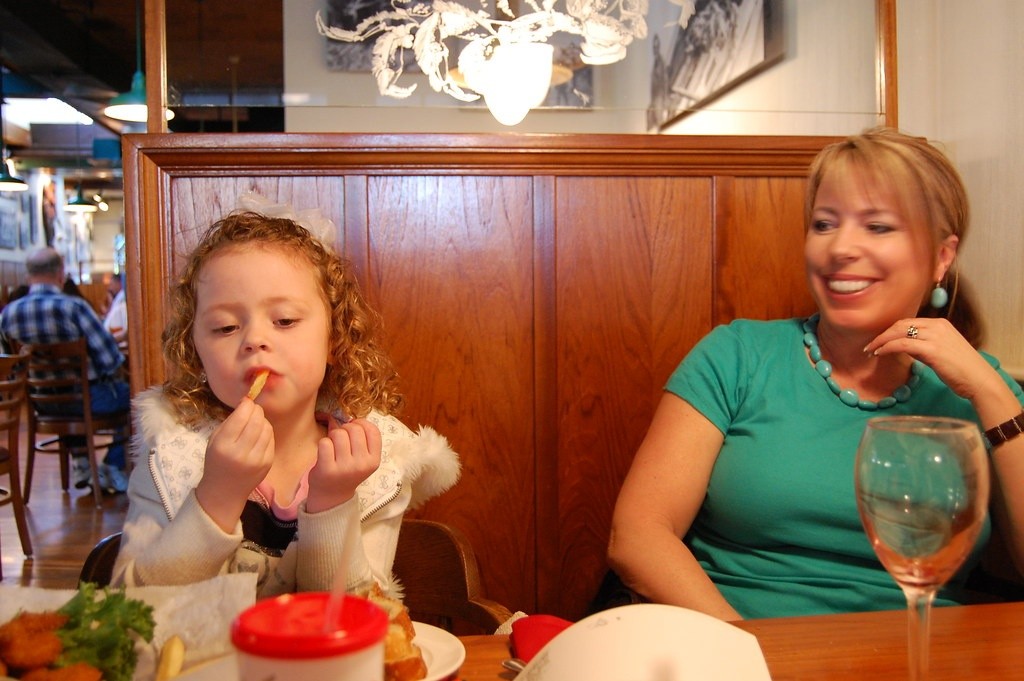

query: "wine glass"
left=856, top=414, right=989, bottom=681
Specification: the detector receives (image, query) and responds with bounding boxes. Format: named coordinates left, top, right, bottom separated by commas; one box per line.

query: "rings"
left=906, top=326, right=919, bottom=340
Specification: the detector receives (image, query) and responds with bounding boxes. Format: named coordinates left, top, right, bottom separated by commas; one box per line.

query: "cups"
left=229, top=594, right=392, bottom=681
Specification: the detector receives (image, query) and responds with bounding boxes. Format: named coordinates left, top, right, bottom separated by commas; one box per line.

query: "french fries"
left=248, top=370, right=269, bottom=400
left=157, top=635, right=186, bottom=681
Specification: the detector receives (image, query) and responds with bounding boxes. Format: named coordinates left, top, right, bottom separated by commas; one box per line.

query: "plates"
left=411, top=619, right=466, bottom=681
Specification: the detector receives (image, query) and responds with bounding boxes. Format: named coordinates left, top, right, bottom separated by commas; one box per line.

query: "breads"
left=345, top=583, right=428, bottom=681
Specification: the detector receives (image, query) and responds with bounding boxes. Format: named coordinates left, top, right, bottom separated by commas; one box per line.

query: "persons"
left=583, top=118, right=1024, bottom=623
left=108, top=188, right=464, bottom=598
left=0, top=246, right=130, bottom=493
left=103, top=286, right=128, bottom=349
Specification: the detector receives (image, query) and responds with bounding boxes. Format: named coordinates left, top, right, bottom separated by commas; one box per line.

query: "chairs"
left=9, top=336, right=135, bottom=507
left=77, top=520, right=513, bottom=640
left=0, top=345, right=34, bottom=560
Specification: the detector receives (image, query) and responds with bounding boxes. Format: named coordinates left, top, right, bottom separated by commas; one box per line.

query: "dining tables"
left=451, top=602, right=1024, bottom=681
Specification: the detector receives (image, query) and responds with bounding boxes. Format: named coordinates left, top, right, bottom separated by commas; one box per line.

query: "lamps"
left=103, top=0, right=178, bottom=123
left=65, top=118, right=98, bottom=212
left=1, top=102, right=29, bottom=190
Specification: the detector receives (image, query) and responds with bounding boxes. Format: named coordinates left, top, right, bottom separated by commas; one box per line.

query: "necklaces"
left=803, top=312, right=924, bottom=409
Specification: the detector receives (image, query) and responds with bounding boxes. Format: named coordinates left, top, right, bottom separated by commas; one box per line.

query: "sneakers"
left=89, top=462, right=128, bottom=493
left=72, top=456, right=91, bottom=488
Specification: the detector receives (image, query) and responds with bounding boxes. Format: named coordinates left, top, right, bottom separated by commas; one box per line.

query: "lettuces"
left=53, top=580, right=158, bottom=681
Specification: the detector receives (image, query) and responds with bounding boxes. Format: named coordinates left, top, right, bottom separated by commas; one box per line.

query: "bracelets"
left=982, top=409, right=1024, bottom=450
